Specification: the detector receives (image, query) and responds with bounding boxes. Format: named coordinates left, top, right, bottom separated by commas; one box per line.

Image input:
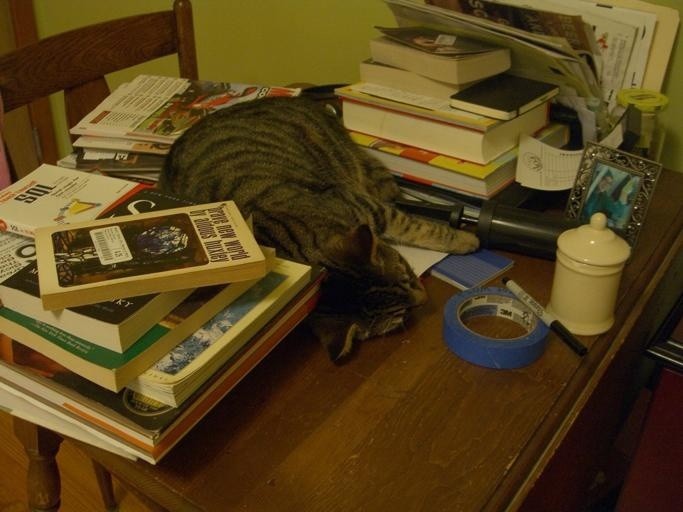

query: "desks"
left=59, top=154, right=683, bottom=512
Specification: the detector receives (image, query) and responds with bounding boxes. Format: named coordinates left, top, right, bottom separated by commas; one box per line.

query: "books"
left=429, top=248, right=515, bottom=291
left=0, top=161, right=328, bottom=466
left=333, top=0, right=683, bottom=221
left=57, top=73, right=302, bottom=189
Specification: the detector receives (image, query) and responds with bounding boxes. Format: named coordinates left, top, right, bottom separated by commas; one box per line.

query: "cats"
left=155, top=94, right=481, bottom=364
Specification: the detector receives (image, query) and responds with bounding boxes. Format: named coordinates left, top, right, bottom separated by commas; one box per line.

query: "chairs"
left=1, top=0, right=199, bottom=512
left=610, top=339, right=683, bottom=512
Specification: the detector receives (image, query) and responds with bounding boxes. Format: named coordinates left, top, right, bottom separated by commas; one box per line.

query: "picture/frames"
left=563, top=141, right=663, bottom=265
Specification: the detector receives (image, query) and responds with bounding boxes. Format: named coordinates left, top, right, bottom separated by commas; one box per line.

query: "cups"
left=547, top=213, right=630, bottom=334
left=621, top=90, right=670, bottom=162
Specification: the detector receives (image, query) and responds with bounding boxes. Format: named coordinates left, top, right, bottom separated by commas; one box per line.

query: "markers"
left=502, top=277, right=588, bottom=357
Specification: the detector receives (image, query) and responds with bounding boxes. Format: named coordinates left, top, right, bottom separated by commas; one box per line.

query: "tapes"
left=444, top=287, right=550, bottom=368
